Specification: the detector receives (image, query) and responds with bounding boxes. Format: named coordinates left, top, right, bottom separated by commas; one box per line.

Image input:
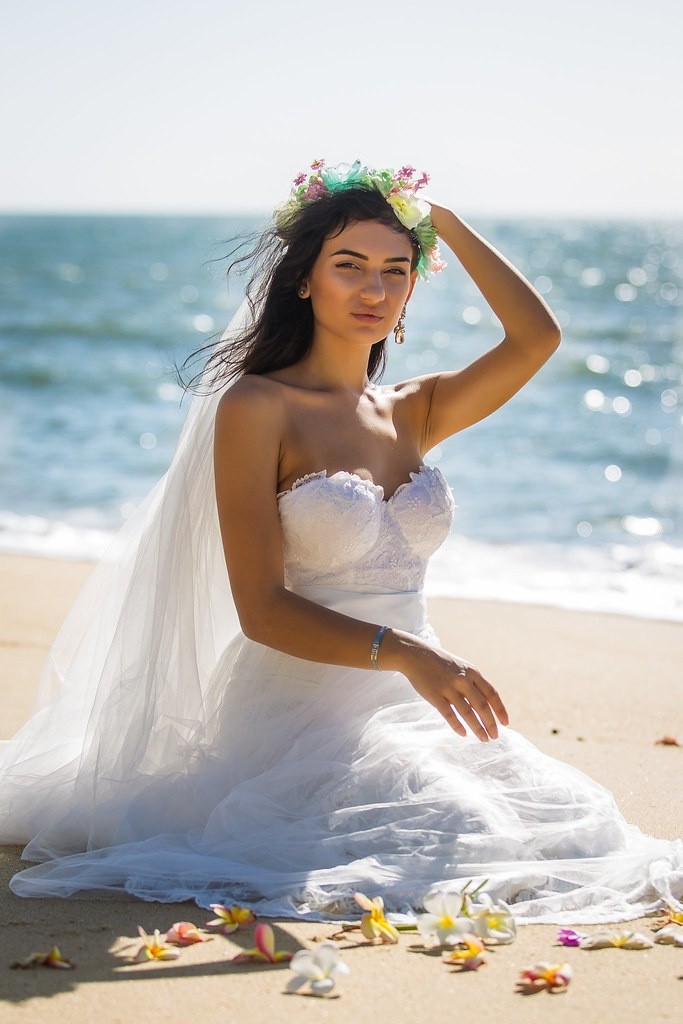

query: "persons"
left=211, top=157, right=619, bottom=866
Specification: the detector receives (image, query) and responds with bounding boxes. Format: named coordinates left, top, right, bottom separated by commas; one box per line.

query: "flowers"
left=293, top=164, right=445, bottom=280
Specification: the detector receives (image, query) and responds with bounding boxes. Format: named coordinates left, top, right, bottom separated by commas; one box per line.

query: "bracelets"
left=370, top=624, right=390, bottom=671
